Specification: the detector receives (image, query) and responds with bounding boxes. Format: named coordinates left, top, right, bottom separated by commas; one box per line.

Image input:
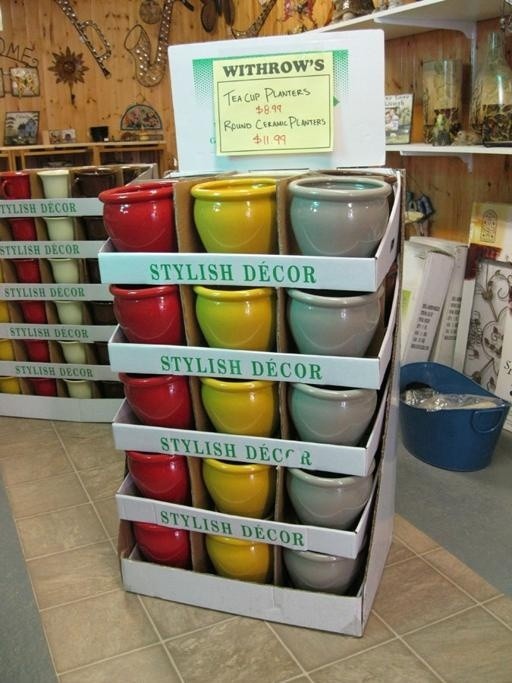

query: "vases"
left=0, top=169, right=125, bottom=399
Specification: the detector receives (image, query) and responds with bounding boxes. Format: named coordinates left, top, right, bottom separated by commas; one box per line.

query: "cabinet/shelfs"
left=0, top=141, right=167, bottom=179
left=304, top=0, right=512, bottom=172
